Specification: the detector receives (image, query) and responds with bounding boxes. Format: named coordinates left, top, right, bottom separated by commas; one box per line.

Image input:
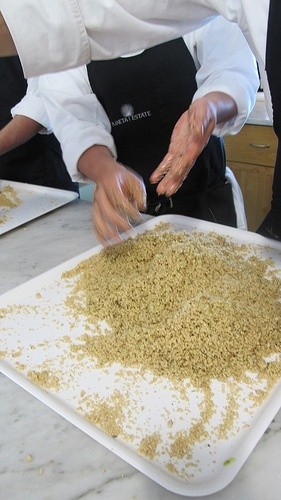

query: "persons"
left=0, top=7, right=88, bottom=198
left=39, top=15, right=261, bottom=249
left=0, top=0, right=281, bottom=246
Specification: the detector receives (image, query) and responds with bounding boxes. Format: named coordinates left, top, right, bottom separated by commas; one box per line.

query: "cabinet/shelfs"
left=222, top=120, right=278, bottom=232
